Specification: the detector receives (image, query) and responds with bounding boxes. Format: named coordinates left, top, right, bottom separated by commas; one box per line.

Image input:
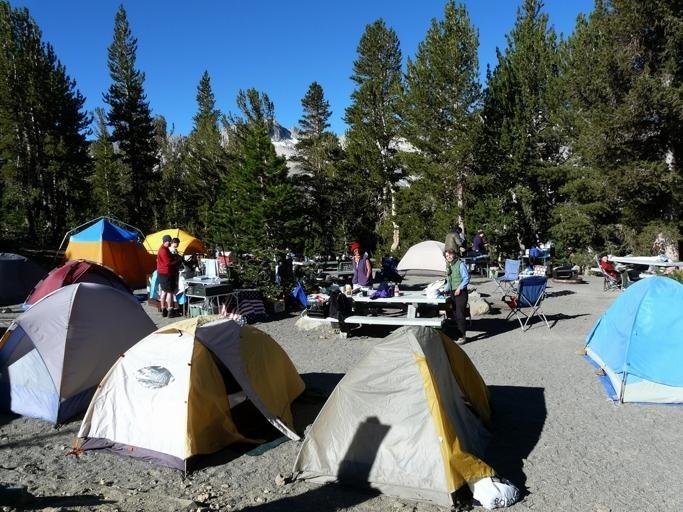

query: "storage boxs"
left=326, top=315, right=446, bottom=327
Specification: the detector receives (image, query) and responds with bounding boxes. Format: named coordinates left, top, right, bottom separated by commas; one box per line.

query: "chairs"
left=454, top=336, right=466, bottom=344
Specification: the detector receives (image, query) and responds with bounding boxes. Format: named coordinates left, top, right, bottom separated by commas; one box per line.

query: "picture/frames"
left=461, top=256, right=490, bottom=279
left=183, top=275, right=241, bottom=318
left=340, top=290, right=452, bottom=340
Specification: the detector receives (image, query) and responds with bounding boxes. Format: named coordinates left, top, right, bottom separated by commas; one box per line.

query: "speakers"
left=162, top=307, right=181, bottom=318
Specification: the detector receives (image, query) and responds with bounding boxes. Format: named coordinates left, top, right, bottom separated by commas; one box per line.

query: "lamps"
left=501, top=276, right=551, bottom=331
left=595, top=253, right=629, bottom=292
left=492, top=258, right=522, bottom=296
left=514, top=264, right=548, bottom=301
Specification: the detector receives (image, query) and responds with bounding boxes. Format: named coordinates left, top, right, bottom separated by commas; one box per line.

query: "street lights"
left=329, top=293, right=352, bottom=317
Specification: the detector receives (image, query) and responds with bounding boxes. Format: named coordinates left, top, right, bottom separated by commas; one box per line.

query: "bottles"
left=164, top=236, right=171, bottom=241
left=351, top=243, right=360, bottom=252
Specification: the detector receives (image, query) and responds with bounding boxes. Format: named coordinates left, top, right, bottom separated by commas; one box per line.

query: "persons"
left=598, top=254, right=620, bottom=281
left=442, top=248, right=471, bottom=345
left=350, top=240, right=373, bottom=291
left=170, top=238, right=179, bottom=253
left=156, top=235, right=178, bottom=318
left=444, top=226, right=467, bottom=256
left=473, top=231, right=488, bottom=256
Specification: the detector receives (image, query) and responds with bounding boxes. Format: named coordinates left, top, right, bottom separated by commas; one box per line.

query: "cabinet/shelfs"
left=394, top=284, right=399, bottom=296
